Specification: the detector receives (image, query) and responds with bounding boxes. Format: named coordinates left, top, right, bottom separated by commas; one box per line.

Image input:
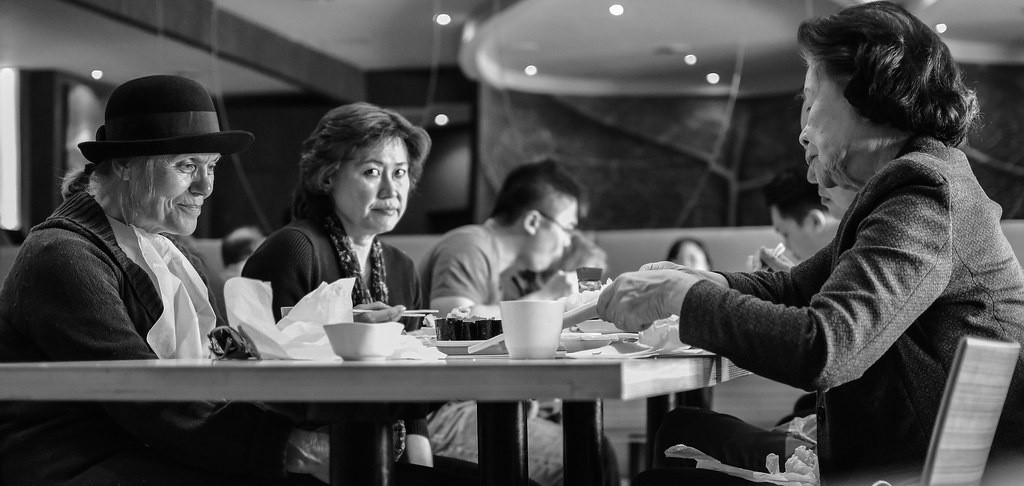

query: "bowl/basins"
left=327, top=323, right=404, bottom=360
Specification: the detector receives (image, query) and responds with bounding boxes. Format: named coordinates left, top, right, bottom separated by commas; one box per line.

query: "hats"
left=76, top=75, right=257, bottom=162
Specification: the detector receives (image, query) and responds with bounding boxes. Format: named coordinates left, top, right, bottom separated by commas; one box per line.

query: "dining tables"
left=0, top=346, right=756, bottom=486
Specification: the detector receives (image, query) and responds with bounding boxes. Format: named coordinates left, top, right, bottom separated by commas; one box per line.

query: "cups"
left=500, top=300, right=565, bottom=359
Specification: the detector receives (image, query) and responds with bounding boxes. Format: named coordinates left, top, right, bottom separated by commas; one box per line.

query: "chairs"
left=919, top=331, right=1021, bottom=486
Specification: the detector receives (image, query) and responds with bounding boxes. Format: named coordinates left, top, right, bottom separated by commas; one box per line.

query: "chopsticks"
left=762, top=242, right=785, bottom=271
left=352, top=308, right=439, bottom=317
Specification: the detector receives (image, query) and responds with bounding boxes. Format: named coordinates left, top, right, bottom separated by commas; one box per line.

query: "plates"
left=561, top=333, right=638, bottom=351
left=468, top=296, right=599, bottom=352
left=576, top=318, right=625, bottom=335
left=435, top=340, right=506, bottom=355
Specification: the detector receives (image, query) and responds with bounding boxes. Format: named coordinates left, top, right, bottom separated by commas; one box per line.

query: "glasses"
left=206, top=324, right=262, bottom=361
left=539, top=209, right=579, bottom=240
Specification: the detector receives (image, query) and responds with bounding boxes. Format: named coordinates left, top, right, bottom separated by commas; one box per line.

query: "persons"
left=664, top=236, right=712, bottom=276
left=741, top=160, right=841, bottom=275
left=596, top=0, right=1024, bottom=486
left=0, top=74, right=406, bottom=485
left=217, top=222, right=264, bottom=291
left=501, top=231, right=624, bottom=486
left=244, top=101, right=547, bottom=486
left=416, top=158, right=628, bottom=486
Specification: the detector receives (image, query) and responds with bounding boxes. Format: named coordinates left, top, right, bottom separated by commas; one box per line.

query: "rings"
left=613, top=316, right=625, bottom=327
